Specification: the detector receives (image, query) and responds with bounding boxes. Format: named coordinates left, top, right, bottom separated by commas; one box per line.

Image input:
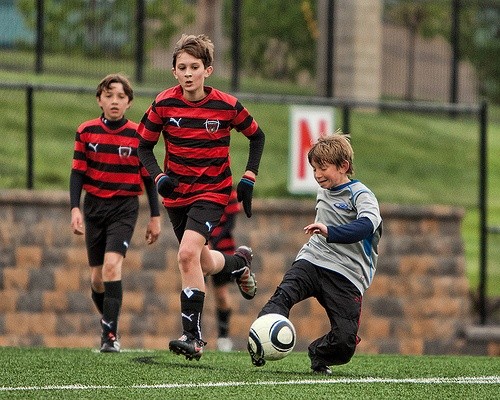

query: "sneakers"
left=247, top=341, right=266, bottom=367
left=234, top=246, right=257, bottom=299
left=100, top=339, right=120, bottom=352
left=169, top=332, right=207, bottom=361
left=314, top=365, right=332, bottom=376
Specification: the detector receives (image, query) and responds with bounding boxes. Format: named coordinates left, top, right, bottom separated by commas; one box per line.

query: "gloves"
left=237, top=175, right=256, bottom=218
left=155, top=174, right=178, bottom=198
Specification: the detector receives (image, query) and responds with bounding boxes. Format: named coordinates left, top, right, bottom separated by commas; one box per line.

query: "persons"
left=133, top=34, right=266, bottom=362
left=248, top=131, right=384, bottom=375
left=69, top=73, right=161, bottom=353
left=202, top=186, right=241, bottom=353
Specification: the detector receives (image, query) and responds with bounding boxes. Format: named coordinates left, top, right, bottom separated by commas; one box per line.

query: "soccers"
left=248, top=313, right=296, bottom=361
